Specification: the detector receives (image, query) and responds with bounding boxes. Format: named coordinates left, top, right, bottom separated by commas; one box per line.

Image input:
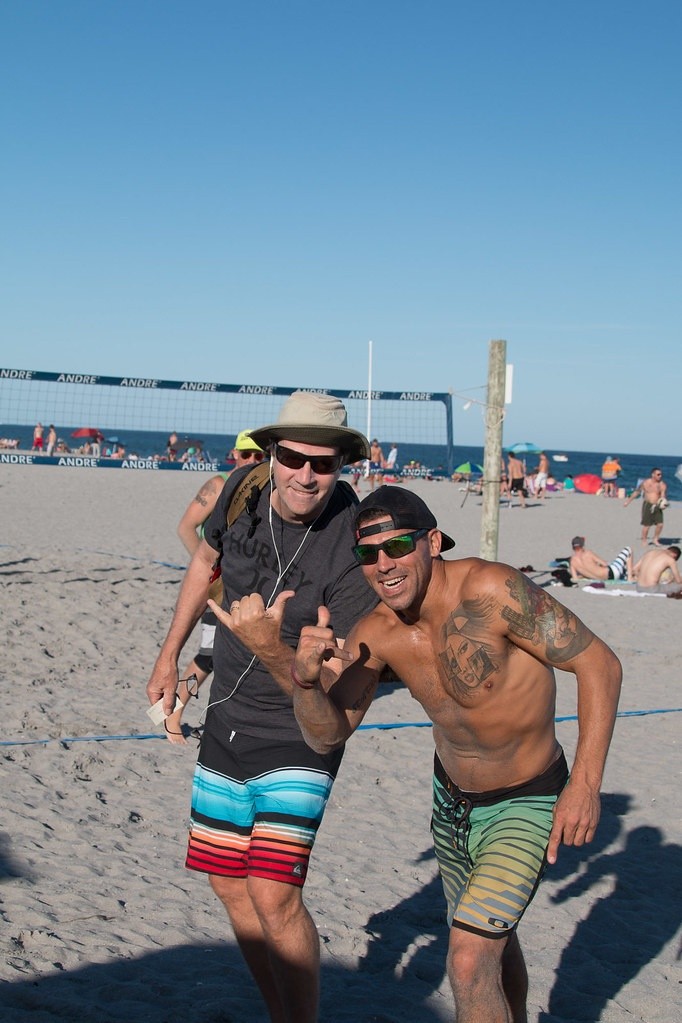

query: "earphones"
left=269, top=452, right=276, bottom=468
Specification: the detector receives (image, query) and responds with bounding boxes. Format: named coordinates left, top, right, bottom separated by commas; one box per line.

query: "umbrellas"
left=105, top=436, right=127, bottom=453
left=454, top=461, right=484, bottom=475
left=72, top=427, right=101, bottom=437
left=503, top=442, right=545, bottom=474
left=574, top=473, right=605, bottom=494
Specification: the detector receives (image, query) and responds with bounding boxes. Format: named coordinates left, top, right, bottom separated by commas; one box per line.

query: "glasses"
left=240, top=451, right=265, bottom=462
left=275, top=441, right=343, bottom=475
left=351, top=529, right=429, bottom=565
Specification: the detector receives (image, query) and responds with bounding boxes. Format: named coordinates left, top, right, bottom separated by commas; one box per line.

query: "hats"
left=233, top=429, right=264, bottom=451
left=350, top=485, right=455, bottom=553
left=245, top=391, right=372, bottom=466
left=572, top=536, right=584, bottom=547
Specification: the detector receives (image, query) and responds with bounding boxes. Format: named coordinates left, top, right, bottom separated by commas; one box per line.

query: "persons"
left=30, top=422, right=44, bottom=456
left=530, top=451, right=549, bottom=501
left=506, top=451, right=527, bottom=509
left=403, top=461, right=445, bottom=481
left=294, top=484, right=623, bottom=1023
left=501, top=458, right=505, bottom=471
left=601, top=455, right=622, bottom=498
left=57, top=430, right=126, bottom=459
left=452, top=473, right=575, bottom=498
left=632, top=546, right=682, bottom=594
left=623, top=468, right=667, bottom=547
left=161, top=429, right=269, bottom=745
left=383, top=442, right=397, bottom=480
left=147, top=391, right=381, bottom=1023
left=45, top=425, right=57, bottom=456
left=570, top=536, right=636, bottom=582
left=366, top=439, right=388, bottom=492
left=129, top=430, right=209, bottom=464
left=350, top=461, right=362, bottom=493
left=0, top=436, right=21, bottom=450
